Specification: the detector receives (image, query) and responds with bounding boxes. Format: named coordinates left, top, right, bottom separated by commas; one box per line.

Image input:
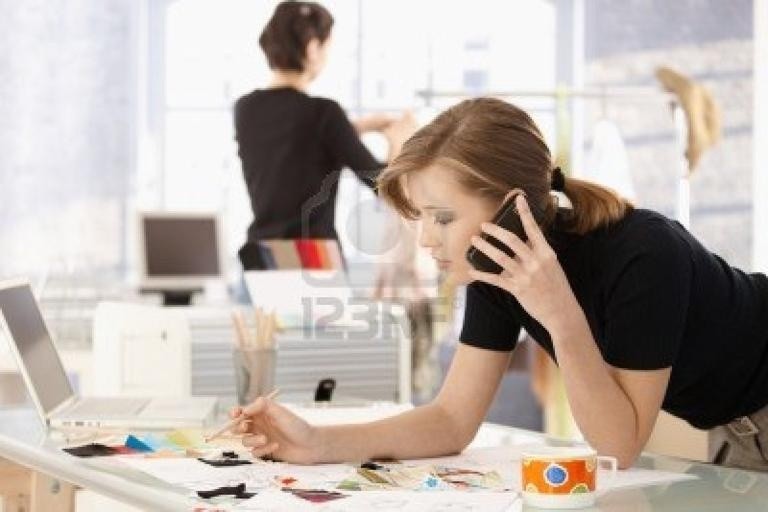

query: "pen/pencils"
left=230, top=306, right=278, bottom=405
left=204, top=387, right=281, bottom=443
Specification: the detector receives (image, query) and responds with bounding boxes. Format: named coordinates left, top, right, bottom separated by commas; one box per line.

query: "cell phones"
left=466, top=193, right=546, bottom=275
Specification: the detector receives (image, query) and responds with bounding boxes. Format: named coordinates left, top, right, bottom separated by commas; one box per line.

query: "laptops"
left=0, top=276, right=221, bottom=432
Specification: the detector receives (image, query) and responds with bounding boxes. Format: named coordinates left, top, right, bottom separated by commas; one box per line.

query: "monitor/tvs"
left=140, top=214, right=221, bottom=308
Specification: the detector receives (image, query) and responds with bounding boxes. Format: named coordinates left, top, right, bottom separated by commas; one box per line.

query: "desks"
left=0, top=405, right=768, bottom=512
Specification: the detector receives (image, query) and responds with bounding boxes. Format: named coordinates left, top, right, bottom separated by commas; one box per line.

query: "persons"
left=229, top=96, right=768, bottom=475
left=232, top=1, right=422, bottom=273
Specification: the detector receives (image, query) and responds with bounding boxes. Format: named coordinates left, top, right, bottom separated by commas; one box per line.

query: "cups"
left=521, top=448, right=617, bottom=509
left=233, top=342, right=277, bottom=407
